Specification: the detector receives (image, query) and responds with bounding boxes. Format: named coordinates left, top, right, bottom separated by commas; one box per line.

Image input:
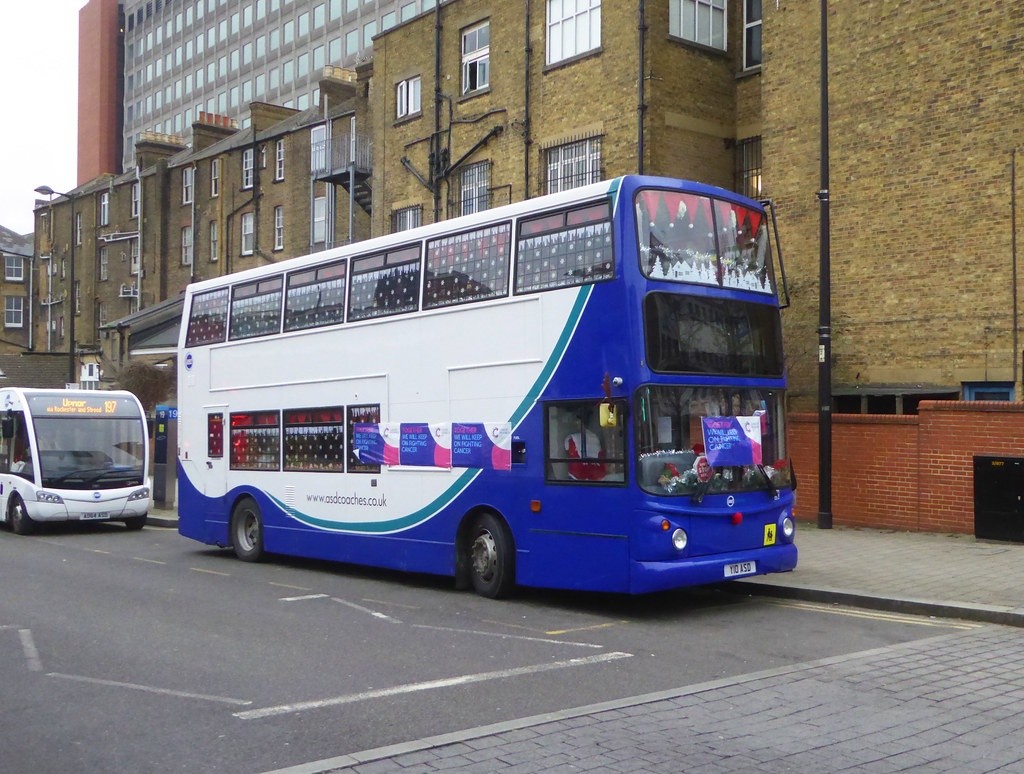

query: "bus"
left=174, top=175, right=798, bottom=602
left=0, top=386, right=164, bottom=531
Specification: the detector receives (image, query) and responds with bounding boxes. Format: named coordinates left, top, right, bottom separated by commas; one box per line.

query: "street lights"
left=35, top=186, right=76, bottom=386
